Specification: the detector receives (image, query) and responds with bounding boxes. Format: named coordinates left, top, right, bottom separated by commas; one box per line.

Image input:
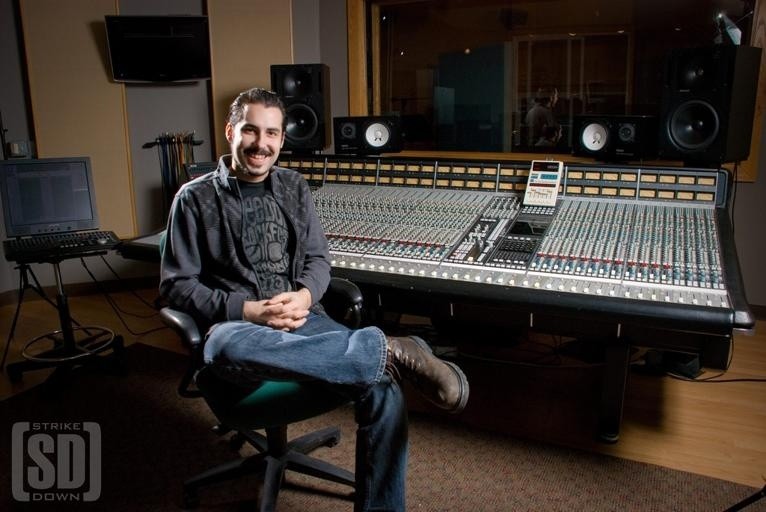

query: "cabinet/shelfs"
left=506, top=30, right=638, bottom=154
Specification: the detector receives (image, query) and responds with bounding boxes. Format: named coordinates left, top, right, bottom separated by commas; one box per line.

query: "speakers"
left=660, top=43, right=761, bottom=169
left=569, top=113, right=659, bottom=164
left=269, top=63, right=332, bottom=158
left=333, top=115, right=406, bottom=156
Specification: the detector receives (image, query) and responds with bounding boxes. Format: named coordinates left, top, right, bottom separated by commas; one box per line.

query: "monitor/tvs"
left=103, top=14, right=213, bottom=85
left=0, top=156, right=100, bottom=238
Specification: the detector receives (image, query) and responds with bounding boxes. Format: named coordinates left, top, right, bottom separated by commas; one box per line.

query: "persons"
left=159, top=88, right=470, bottom=512
left=525, top=84, right=563, bottom=148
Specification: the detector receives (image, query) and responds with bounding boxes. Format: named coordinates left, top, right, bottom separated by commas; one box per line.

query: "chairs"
left=155, top=226, right=364, bottom=512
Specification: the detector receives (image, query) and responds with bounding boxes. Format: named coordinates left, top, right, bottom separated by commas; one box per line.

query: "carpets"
left=0, top=342, right=766, bottom=512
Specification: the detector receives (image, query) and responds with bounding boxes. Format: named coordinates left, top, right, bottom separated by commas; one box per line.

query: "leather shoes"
left=384, top=335, right=470, bottom=412
